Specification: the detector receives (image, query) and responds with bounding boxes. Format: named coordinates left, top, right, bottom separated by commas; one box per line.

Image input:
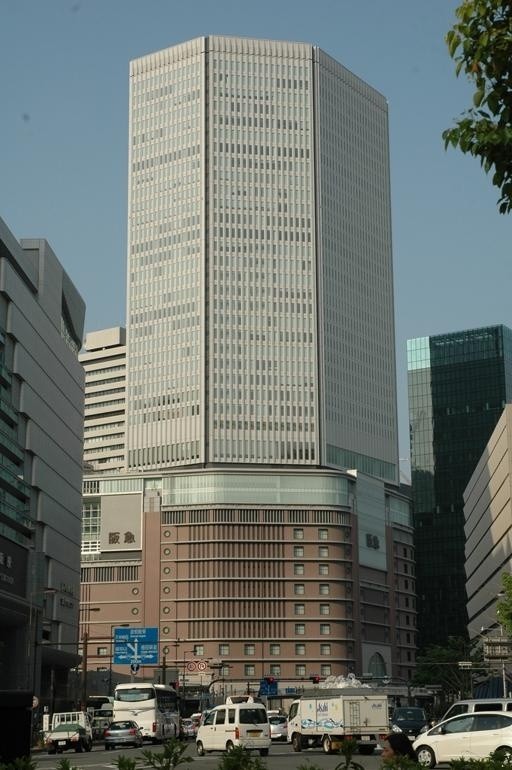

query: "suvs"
left=389, top=707, right=428, bottom=737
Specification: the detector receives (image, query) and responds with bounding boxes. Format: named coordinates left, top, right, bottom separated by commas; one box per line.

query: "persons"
left=381, top=733, right=418, bottom=765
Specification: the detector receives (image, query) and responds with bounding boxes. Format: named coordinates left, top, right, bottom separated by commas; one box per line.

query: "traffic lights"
left=267, top=677, right=274, bottom=686
left=313, top=677, right=320, bottom=684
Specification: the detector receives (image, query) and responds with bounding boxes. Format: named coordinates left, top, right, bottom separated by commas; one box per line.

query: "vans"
left=194, top=703, right=272, bottom=758
left=437, top=698, right=512, bottom=733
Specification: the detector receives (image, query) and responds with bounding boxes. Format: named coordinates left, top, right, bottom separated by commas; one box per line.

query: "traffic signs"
left=113, top=626, right=159, bottom=666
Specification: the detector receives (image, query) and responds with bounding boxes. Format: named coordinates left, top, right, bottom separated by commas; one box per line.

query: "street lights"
left=74, top=607, right=101, bottom=712
left=108, top=622, right=131, bottom=697
left=182, top=649, right=198, bottom=718
left=161, top=643, right=180, bottom=684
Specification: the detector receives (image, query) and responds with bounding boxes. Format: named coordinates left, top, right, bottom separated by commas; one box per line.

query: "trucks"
left=44, top=711, right=93, bottom=747
left=286, top=691, right=389, bottom=755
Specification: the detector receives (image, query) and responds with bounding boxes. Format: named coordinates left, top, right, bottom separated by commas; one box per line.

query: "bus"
left=113, top=683, right=180, bottom=745
left=85, top=696, right=114, bottom=736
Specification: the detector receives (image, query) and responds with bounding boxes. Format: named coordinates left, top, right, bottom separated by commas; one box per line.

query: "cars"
left=412, top=710, right=512, bottom=769
left=181, top=695, right=287, bottom=740
left=103, top=720, right=144, bottom=750
left=45, top=723, right=93, bottom=755
